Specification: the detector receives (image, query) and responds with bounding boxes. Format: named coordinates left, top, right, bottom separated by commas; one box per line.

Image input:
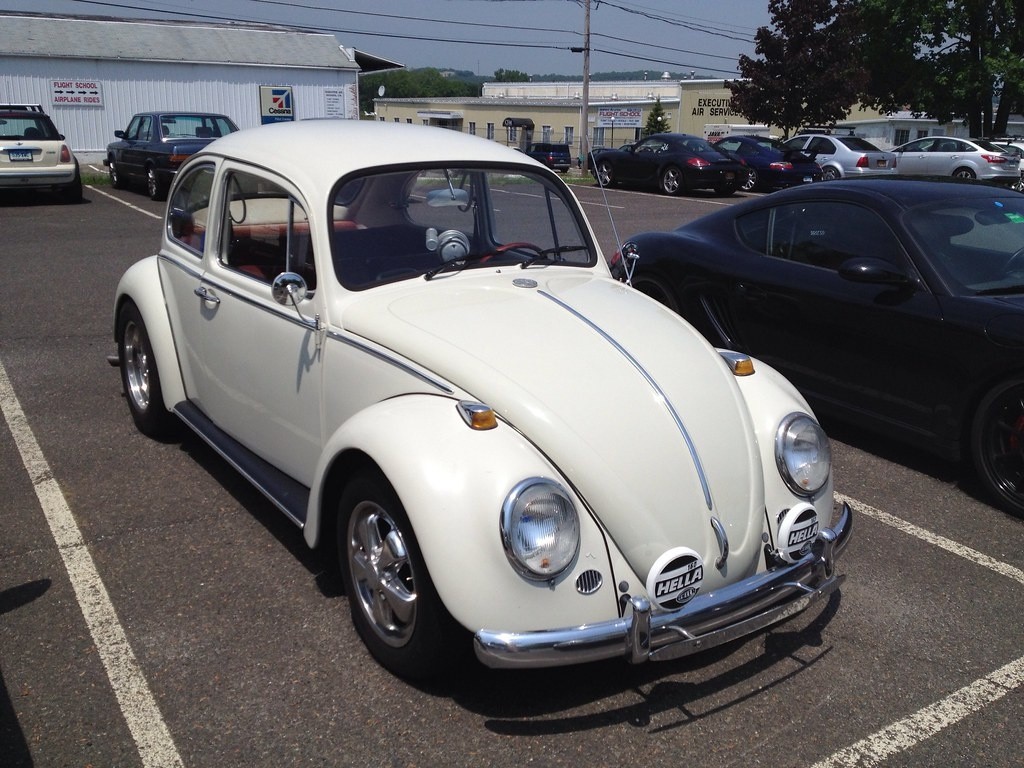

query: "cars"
left=608, top=177, right=1023, bottom=516
left=780, top=133, right=896, bottom=182
left=0, top=102, right=83, bottom=200
left=102, top=110, right=241, bottom=201
left=987, top=140, right=1024, bottom=173
left=591, top=132, right=746, bottom=198
left=104, top=119, right=853, bottom=675
left=710, top=134, right=823, bottom=192
left=886, top=135, right=1021, bottom=188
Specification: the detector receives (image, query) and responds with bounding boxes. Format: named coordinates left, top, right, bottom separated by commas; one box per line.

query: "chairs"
left=908, top=212, right=985, bottom=287
left=940, top=143, right=957, bottom=152
left=25, top=127, right=42, bottom=138
left=196, top=127, right=212, bottom=138
left=160, top=125, right=169, bottom=137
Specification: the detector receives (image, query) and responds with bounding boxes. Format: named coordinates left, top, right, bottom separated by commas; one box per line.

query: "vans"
left=525, top=141, right=572, bottom=173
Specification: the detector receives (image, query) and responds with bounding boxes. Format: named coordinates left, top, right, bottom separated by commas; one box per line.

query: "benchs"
left=180, top=220, right=356, bottom=265
left=231, top=250, right=476, bottom=290
